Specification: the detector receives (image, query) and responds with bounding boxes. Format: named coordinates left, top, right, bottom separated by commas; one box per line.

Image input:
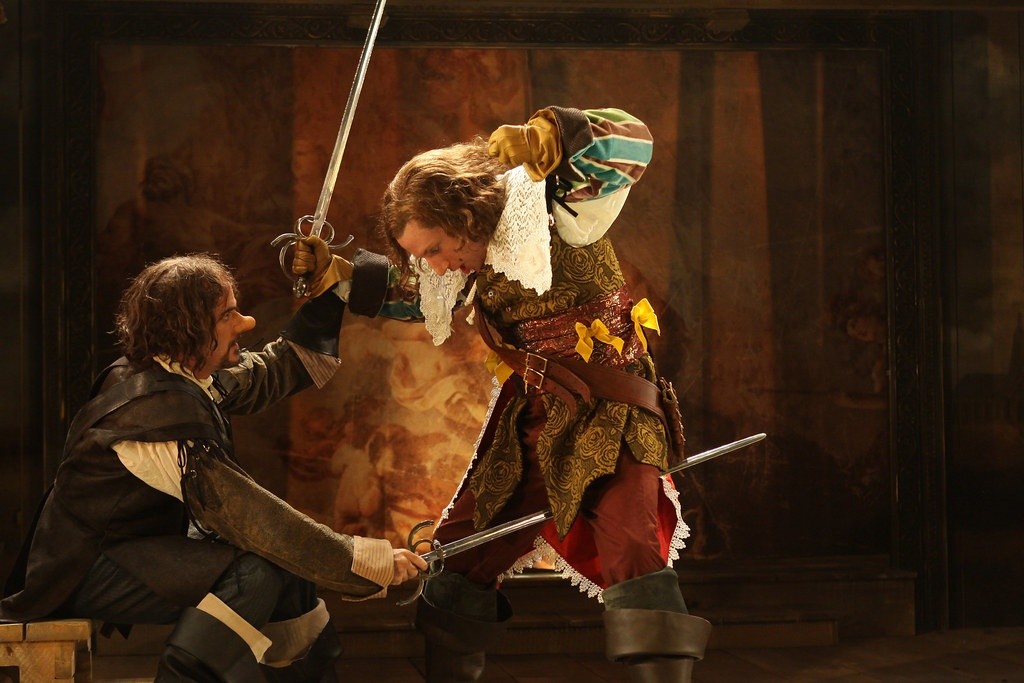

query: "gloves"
left=485, top=109, right=563, bottom=182
left=293, top=236, right=355, bottom=300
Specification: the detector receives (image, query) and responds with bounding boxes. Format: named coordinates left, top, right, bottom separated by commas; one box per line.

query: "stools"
left=0, top=620, right=93, bottom=683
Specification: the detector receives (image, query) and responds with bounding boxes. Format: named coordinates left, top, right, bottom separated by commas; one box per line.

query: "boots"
left=603, top=609, right=711, bottom=682
left=263, top=599, right=346, bottom=682
left=414, top=587, right=512, bottom=682
left=156, top=606, right=269, bottom=682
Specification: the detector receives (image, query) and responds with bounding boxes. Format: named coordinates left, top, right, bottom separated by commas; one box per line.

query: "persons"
left=292, top=106, right=711, bottom=683
left=0, top=250, right=428, bottom=683
